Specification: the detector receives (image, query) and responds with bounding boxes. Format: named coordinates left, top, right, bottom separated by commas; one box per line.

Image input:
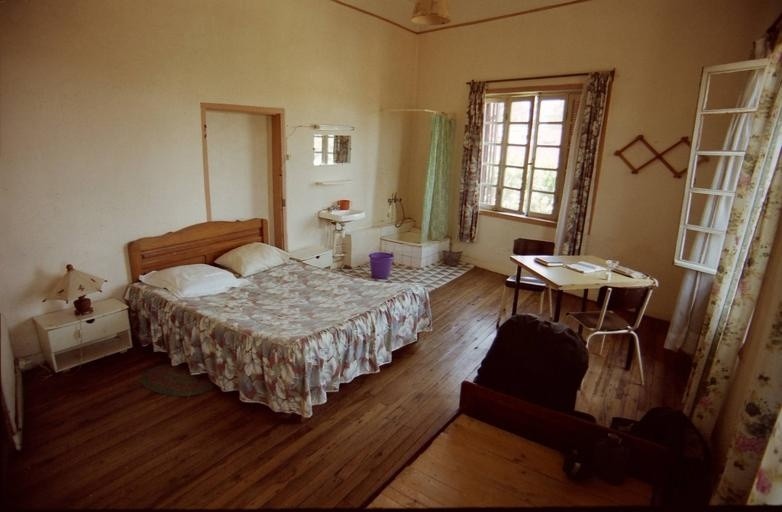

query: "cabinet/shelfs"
left=31, top=297, right=133, bottom=375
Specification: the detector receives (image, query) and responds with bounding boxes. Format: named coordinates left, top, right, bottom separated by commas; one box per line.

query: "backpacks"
left=630, top=407, right=710, bottom=467
left=473, top=312, right=589, bottom=416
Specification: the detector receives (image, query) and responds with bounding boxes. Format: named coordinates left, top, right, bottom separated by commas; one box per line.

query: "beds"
left=126, top=217, right=435, bottom=418
left=356, top=376, right=674, bottom=508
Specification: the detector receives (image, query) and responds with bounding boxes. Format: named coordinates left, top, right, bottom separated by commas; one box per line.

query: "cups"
left=337, top=200, right=350, bottom=210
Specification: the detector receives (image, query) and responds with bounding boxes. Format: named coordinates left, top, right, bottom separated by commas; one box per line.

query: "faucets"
left=328, top=205, right=341, bottom=212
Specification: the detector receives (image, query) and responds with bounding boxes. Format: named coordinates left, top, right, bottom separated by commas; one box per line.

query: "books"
left=534, top=255, right=564, bottom=267
left=611, top=264, right=647, bottom=280
left=565, top=260, right=605, bottom=274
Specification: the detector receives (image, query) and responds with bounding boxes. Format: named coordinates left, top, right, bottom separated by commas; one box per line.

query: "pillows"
left=134, top=261, right=243, bottom=300
left=214, top=241, right=293, bottom=278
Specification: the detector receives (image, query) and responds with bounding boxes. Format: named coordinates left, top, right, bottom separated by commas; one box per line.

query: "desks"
left=503, top=254, right=661, bottom=372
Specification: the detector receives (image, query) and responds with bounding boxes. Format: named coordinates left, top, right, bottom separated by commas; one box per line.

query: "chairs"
left=494, top=237, right=560, bottom=327
left=566, top=281, right=658, bottom=387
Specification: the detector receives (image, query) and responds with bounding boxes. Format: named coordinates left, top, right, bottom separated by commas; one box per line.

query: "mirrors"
left=312, top=133, right=352, bottom=165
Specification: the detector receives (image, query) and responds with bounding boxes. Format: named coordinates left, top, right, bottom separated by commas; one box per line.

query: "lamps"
left=408, top=0, right=452, bottom=26
left=41, top=263, right=108, bottom=317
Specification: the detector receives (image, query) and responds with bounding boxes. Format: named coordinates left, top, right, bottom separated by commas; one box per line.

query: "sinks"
left=380, top=228, right=450, bottom=246
left=319, top=208, right=366, bottom=222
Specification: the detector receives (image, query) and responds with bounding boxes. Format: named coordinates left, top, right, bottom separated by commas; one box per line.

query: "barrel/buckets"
left=369, top=252, right=394, bottom=278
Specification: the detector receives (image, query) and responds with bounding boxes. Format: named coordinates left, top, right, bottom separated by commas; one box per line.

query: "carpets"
left=139, top=358, right=215, bottom=399
